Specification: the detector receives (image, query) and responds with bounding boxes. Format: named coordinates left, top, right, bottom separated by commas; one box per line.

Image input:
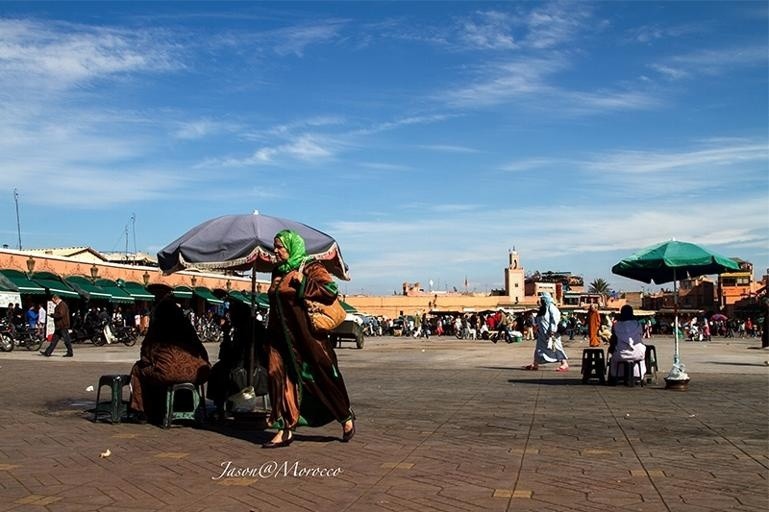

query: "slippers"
left=556, top=366, right=570, bottom=372
left=525, top=364, right=538, bottom=370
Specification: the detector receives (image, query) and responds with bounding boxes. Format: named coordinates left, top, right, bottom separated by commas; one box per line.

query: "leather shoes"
left=64, top=354, right=73, bottom=357
left=40, top=350, right=49, bottom=356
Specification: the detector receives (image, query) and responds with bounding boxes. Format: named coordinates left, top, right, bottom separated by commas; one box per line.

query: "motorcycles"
left=0, top=302, right=229, bottom=355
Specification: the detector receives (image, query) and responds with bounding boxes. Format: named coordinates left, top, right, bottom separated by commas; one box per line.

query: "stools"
left=161, top=382, right=202, bottom=428
left=607, top=351, right=612, bottom=372
left=94, top=375, right=132, bottom=424
left=614, top=359, right=643, bottom=387
left=223, top=393, right=268, bottom=413
left=645, top=345, right=658, bottom=384
left=581, top=349, right=606, bottom=383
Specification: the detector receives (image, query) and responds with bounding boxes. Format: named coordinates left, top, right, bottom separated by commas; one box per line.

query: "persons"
left=361, top=305, right=656, bottom=349
left=606, top=304, right=648, bottom=384
left=127, top=290, right=211, bottom=424
left=206, top=300, right=269, bottom=420
left=658, top=316, right=763, bottom=342
left=6, top=294, right=125, bottom=358
left=259, top=228, right=357, bottom=448
left=522, top=291, right=569, bottom=371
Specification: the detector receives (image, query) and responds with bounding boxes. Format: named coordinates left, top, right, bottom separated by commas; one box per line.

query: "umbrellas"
left=610, top=237, right=741, bottom=366
left=156, top=209, right=352, bottom=390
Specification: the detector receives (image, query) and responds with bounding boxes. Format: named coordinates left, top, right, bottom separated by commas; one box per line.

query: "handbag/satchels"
left=558, top=319, right=570, bottom=335
left=305, top=296, right=348, bottom=335
left=228, top=385, right=256, bottom=417
left=232, top=366, right=270, bottom=395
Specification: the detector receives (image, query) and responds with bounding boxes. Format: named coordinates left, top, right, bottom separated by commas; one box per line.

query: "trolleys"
left=330, top=321, right=364, bottom=351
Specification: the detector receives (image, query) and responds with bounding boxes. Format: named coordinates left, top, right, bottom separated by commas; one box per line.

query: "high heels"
left=264, top=435, right=293, bottom=448
left=343, top=408, right=357, bottom=443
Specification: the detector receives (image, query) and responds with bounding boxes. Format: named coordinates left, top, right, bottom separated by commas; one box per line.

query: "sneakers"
left=121, top=414, right=148, bottom=425
